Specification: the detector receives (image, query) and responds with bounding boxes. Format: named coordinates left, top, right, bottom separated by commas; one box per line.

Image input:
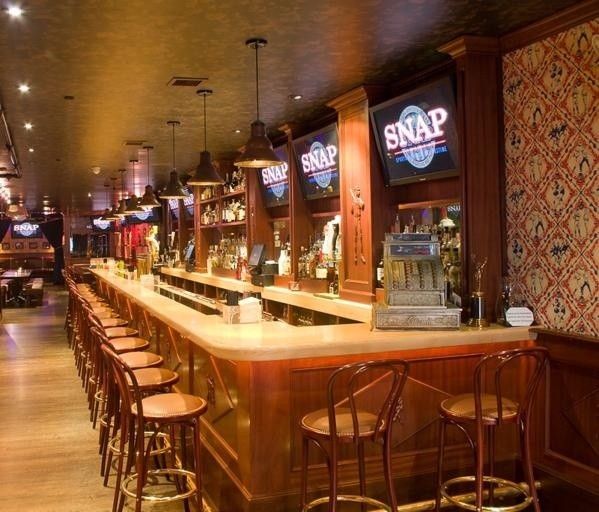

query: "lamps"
left=186, top=90, right=226, bottom=185
left=234, top=39, right=283, bottom=167
left=100, top=121, right=189, bottom=222
left=9, top=205, right=19, bottom=212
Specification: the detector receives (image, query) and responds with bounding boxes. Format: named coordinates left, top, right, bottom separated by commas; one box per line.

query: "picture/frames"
left=2, top=241, right=50, bottom=251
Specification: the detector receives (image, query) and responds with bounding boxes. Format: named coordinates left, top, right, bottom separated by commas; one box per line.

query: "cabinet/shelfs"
left=194, top=168, right=248, bottom=229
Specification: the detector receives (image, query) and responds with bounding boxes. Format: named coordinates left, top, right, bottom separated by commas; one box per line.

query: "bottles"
left=278, top=235, right=340, bottom=278
left=200, top=169, right=249, bottom=282
left=376, top=215, right=464, bottom=307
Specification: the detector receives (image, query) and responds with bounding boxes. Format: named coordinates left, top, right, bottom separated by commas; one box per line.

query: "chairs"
left=60, top=267, right=209, bottom=512
left=298, top=358, right=410, bottom=512
left=435, top=346, right=550, bottom=512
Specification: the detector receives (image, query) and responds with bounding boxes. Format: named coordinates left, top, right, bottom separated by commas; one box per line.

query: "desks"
left=0, top=268, right=33, bottom=302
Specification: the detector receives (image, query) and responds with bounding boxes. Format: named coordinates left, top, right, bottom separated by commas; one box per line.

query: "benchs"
left=22, top=277, right=44, bottom=306
